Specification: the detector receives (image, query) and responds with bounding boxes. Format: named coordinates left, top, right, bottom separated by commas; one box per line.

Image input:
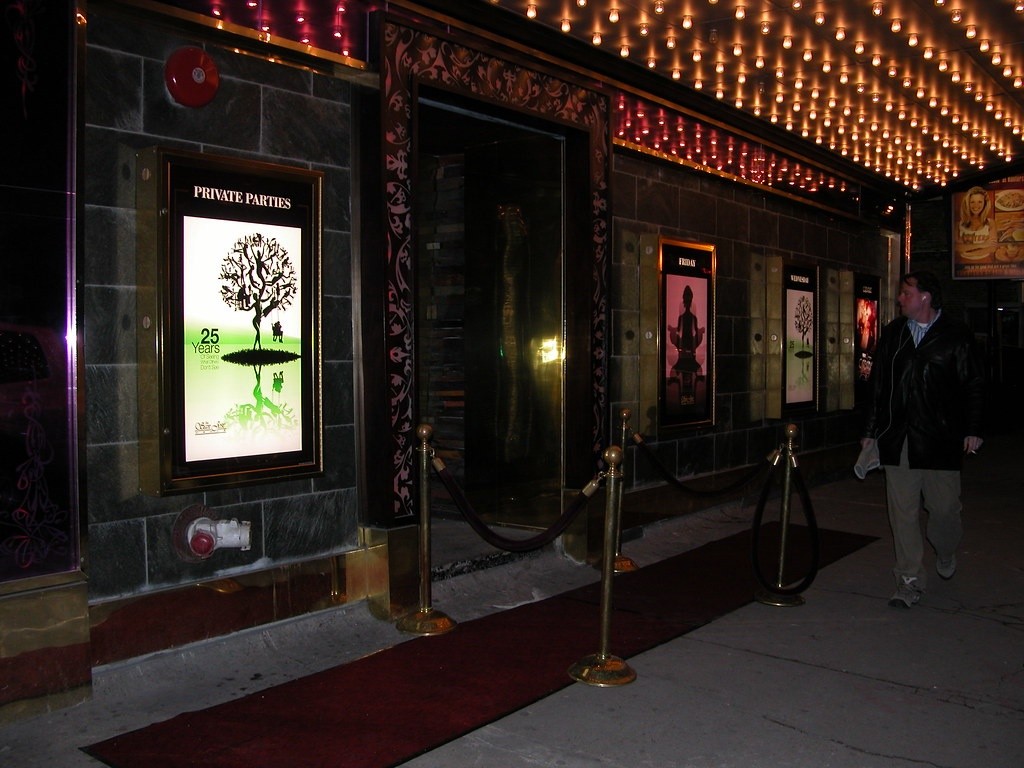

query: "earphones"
left=922, top=296, right=926, bottom=300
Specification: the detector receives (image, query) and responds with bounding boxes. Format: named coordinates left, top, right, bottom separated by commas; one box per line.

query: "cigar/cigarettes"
left=971, top=450, right=976, bottom=454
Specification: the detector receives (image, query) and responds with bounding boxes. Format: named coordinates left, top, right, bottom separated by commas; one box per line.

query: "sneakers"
left=936, top=554, right=958, bottom=580
left=888, top=582, right=926, bottom=609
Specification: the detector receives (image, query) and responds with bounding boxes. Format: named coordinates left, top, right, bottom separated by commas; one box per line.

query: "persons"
left=859, top=271, right=984, bottom=608
